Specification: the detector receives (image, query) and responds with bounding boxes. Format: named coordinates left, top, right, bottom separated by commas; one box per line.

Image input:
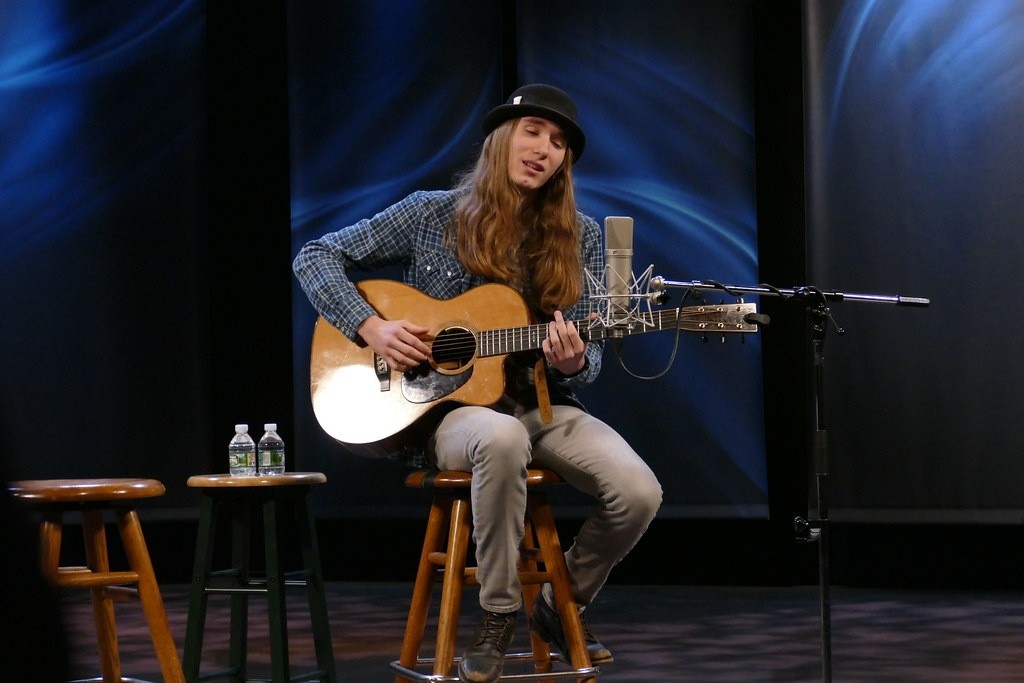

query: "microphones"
left=604, top=216, right=634, bottom=353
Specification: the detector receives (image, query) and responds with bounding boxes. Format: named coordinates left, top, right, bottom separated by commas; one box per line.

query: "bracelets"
left=551, top=353, right=590, bottom=384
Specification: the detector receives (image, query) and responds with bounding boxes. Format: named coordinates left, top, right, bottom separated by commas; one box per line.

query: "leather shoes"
left=456, top=591, right=525, bottom=683
left=529, top=590, right=614, bottom=665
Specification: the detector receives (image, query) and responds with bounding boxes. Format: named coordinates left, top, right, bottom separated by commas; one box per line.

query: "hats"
left=486, top=85, right=585, bottom=163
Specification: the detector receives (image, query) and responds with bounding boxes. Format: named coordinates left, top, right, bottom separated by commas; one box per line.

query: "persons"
left=292, top=84, right=663, bottom=683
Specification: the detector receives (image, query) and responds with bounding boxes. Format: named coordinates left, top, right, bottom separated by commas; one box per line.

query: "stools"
left=9, top=476, right=188, bottom=683
left=389, top=465, right=602, bottom=683
left=182, top=471, right=338, bottom=683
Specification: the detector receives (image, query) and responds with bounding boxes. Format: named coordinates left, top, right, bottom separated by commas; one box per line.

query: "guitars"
left=308, top=278, right=772, bottom=457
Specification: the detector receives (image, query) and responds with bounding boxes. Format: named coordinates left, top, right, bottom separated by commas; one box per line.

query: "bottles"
left=228, top=424, right=256, bottom=477
left=257, top=424, right=285, bottom=476
